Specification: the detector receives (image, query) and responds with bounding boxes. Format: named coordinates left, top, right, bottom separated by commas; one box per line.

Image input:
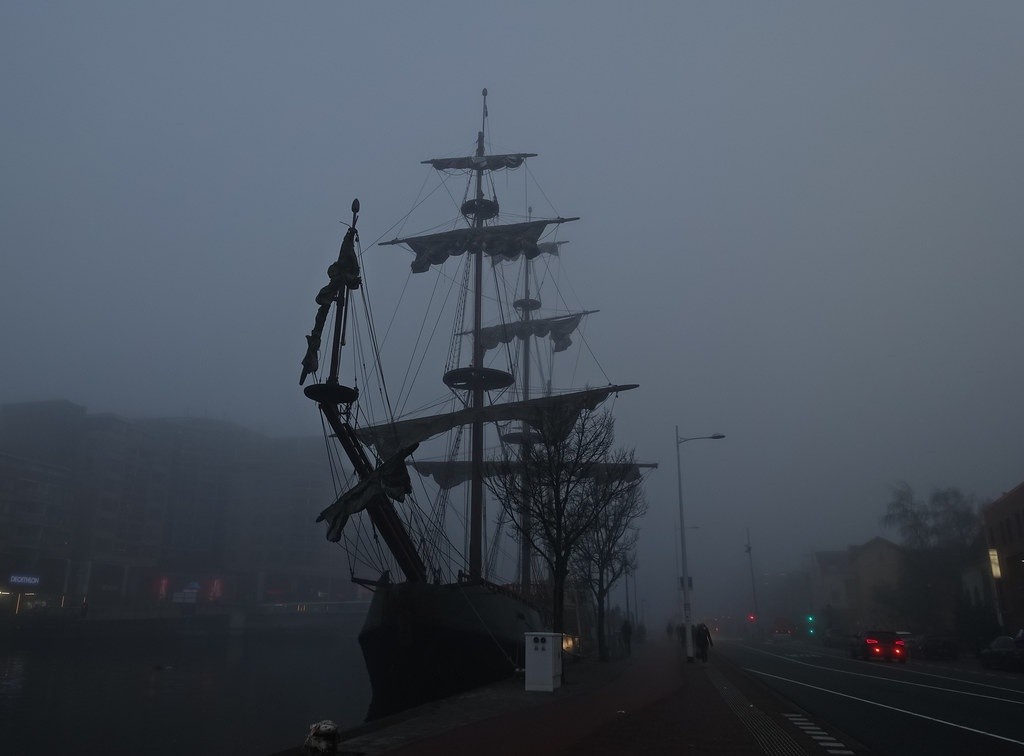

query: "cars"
left=850, top=630, right=908, bottom=664
left=766, top=618, right=792, bottom=642
left=909, top=633, right=957, bottom=660
left=979, top=634, right=1024, bottom=669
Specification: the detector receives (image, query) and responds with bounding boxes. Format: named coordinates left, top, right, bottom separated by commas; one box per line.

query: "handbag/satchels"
left=695, top=652, right=702, bottom=660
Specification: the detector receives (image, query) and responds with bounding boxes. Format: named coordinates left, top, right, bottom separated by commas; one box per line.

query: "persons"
left=667, top=622, right=713, bottom=664
left=620, top=619, right=633, bottom=656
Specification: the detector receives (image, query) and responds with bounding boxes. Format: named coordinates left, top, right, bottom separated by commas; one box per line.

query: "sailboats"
left=299, top=85, right=660, bottom=719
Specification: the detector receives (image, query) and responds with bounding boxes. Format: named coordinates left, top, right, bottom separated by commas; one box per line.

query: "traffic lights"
left=808, top=616, right=815, bottom=635
left=748, top=615, right=754, bottom=621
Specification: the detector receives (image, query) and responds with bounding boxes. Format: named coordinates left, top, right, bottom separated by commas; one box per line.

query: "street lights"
left=674, top=434, right=727, bottom=660
left=674, top=527, right=702, bottom=631
